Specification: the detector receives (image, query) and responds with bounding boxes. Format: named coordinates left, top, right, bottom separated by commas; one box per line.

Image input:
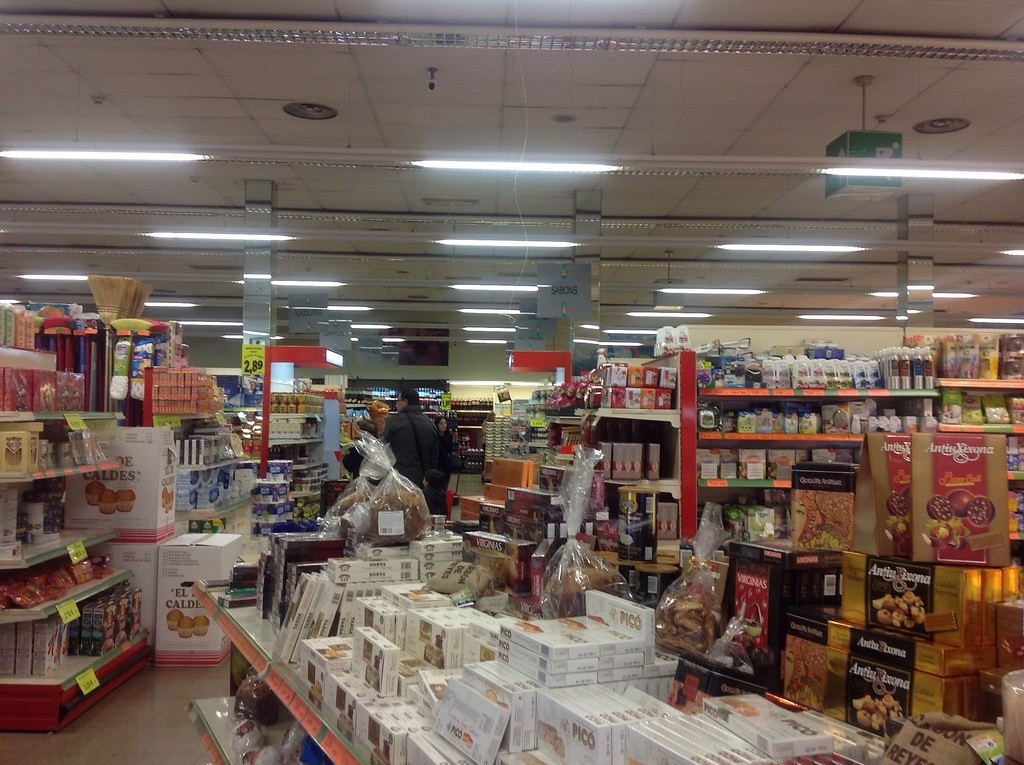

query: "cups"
left=1001, top=669, right=1024, bottom=765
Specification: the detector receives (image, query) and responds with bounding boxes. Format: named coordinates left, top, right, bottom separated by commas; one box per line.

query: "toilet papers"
left=236, top=460, right=294, bottom=534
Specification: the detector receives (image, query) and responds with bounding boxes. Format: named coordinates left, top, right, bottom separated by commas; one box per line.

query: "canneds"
left=271, top=392, right=325, bottom=414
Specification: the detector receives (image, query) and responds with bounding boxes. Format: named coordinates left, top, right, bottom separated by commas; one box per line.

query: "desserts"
left=85, top=480, right=135, bottom=514
left=872, top=591, right=927, bottom=628
left=852, top=694, right=903, bottom=729
left=162, top=487, right=174, bottom=513
left=167, top=609, right=210, bottom=638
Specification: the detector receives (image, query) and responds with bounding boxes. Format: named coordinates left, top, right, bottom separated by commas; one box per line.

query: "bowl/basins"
left=292, top=462, right=329, bottom=493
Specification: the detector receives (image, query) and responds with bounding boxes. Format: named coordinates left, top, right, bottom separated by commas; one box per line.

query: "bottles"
left=450, top=412, right=487, bottom=425
left=449, top=428, right=484, bottom=474
left=451, top=397, right=493, bottom=411
left=531, top=428, right=548, bottom=442
left=370, top=386, right=443, bottom=412
left=874, top=346, right=934, bottom=390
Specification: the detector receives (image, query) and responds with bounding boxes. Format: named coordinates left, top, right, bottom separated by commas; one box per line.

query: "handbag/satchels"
left=438, top=454, right=463, bottom=473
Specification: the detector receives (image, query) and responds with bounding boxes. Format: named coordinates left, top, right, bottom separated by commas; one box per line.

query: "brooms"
left=35, top=276, right=169, bottom=414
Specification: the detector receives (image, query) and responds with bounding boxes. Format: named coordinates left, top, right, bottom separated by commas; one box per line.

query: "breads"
left=652, top=595, right=713, bottom=652
left=544, top=565, right=619, bottom=618
left=337, top=489, right=429, bottom=544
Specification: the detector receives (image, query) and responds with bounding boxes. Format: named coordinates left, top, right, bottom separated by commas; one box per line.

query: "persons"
left=343, top=389, right=462, bottom=515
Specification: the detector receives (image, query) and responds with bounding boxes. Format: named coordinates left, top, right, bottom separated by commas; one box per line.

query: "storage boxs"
left=255, top=488, right=1024, bottom=764
left=86, top=533, right=243, bottom=667
left=64, top=425, right=176, bottom=542
left=0, top=577, right=142, bottom=675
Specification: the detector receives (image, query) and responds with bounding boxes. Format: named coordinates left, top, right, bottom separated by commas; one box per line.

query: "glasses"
left=395, top=399, right=403, bottom=404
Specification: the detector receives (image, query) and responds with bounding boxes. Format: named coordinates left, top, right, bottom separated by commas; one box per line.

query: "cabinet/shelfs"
left=0, top=344, right=154, bottom=733
left=183, top=580, right=371, bottom=765
left=449, top=354, right=1024, bottom=538
left=153, top=405, right=262, bottom=521
left=260, top=344, right=344, bottom=501
left=344, top=397, right=449, bottom=444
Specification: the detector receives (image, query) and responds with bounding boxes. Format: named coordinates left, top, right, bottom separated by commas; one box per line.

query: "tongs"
left=694, top=338, right=750, bottom=353
